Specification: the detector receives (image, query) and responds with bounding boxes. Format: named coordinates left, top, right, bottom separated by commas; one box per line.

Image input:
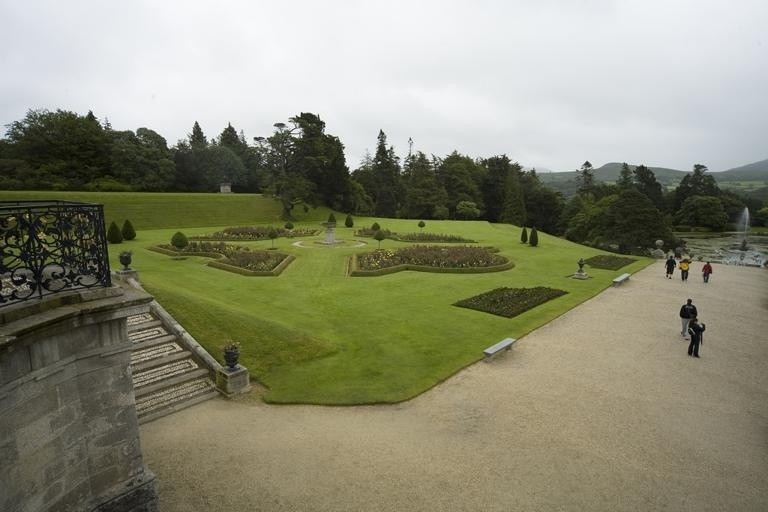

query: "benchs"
left=612, top=272, right=631, bottom=288
left=483, top=337, right=517, bottom=363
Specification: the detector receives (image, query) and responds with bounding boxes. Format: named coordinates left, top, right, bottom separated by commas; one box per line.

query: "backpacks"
left=688, top=328, right=696, bottom=336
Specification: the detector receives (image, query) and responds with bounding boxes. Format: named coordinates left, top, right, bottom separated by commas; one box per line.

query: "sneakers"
left=681, top=331, right=687, bottom=337
left=688, top=353, right=700, bottom=358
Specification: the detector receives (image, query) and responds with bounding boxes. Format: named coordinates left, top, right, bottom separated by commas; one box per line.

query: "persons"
left=679, top=299, right=698, bottom=340
left=664, top=256, right=676, bottom=279
left=702, top=262, right=712, bottom=283
left=679, top=260, right=692, bottom=281
left=688, top=319, right=705, bottom=358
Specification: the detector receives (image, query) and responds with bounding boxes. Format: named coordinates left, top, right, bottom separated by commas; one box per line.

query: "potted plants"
left=116, top=249, right=133, bottom=270
left=221, top=337, right=242, bottom=371
left=576, top=257, right=586, bottom=273
